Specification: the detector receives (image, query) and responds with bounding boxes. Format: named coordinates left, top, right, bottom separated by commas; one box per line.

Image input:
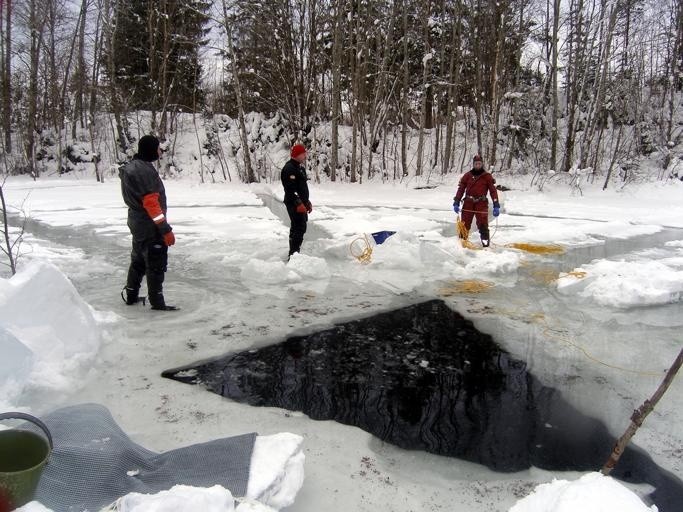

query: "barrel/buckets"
left=0, top=413, right=54, bottom=512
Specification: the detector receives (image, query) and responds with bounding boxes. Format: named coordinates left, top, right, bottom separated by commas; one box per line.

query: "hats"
left=291, top=144, right=305, bottom=158
left=474, top=155, right=482, bottom=162
left=138, top=135, right=159, bottom=162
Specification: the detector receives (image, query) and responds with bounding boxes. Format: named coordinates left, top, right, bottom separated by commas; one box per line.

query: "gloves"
left=162, top=231, right=175, bottom=246
left=297, top=200, right=312, bottom=213
left=454, top=205, right=459, bottom=212
left=493, top=207, right=499, bottom=216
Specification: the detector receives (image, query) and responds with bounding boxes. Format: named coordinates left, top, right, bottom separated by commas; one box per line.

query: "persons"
left=118, top=136, right=179, bottom=311
left=281, top=146, right=312, bottom=261
left=453, top=156, right=500, bottom=247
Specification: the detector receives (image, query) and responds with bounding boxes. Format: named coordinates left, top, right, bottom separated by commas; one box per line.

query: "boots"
left=148, top=294, right=176, bottom=310
left=126, top=287, right=145, bottom=305
